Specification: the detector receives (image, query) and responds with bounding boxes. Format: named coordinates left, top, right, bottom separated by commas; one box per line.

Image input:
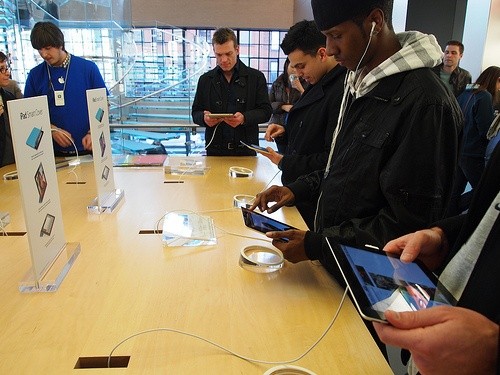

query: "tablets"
left=94, top=107, right=110, bottom=180
left=240, top=140, right=284, bottom=157
left=206, top=113, right=233, bottom=118
left=241, top=206, right=300, bottom=242
left=325, top=236, right=464, bottom=324
left=26, top=126, right=56, bottom=237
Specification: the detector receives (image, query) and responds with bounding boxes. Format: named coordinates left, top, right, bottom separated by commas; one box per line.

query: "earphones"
left=370, top=21, right=376, bottom=37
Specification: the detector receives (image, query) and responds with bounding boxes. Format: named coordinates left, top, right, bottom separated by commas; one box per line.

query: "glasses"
left=0, top=68, right=8, bottom=74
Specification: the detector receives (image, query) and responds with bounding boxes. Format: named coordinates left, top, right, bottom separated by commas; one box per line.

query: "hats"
left=311, top=0, right=378, bottom=30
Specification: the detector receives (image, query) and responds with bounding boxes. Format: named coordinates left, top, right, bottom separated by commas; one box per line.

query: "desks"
left=0, top=156, right=395, bottom=375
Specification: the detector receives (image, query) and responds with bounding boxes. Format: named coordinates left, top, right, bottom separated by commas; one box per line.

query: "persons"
left=0, top=0, right=109, bottom=167
left=192, top=28, right=273, bottom=157
left=431, top=40, right=500, bottom=214
left=248, top=0, right=466, bottom=292
left=372, top=138, right=500, bottom=375
left=261, top=20, right=347, bottom=229
